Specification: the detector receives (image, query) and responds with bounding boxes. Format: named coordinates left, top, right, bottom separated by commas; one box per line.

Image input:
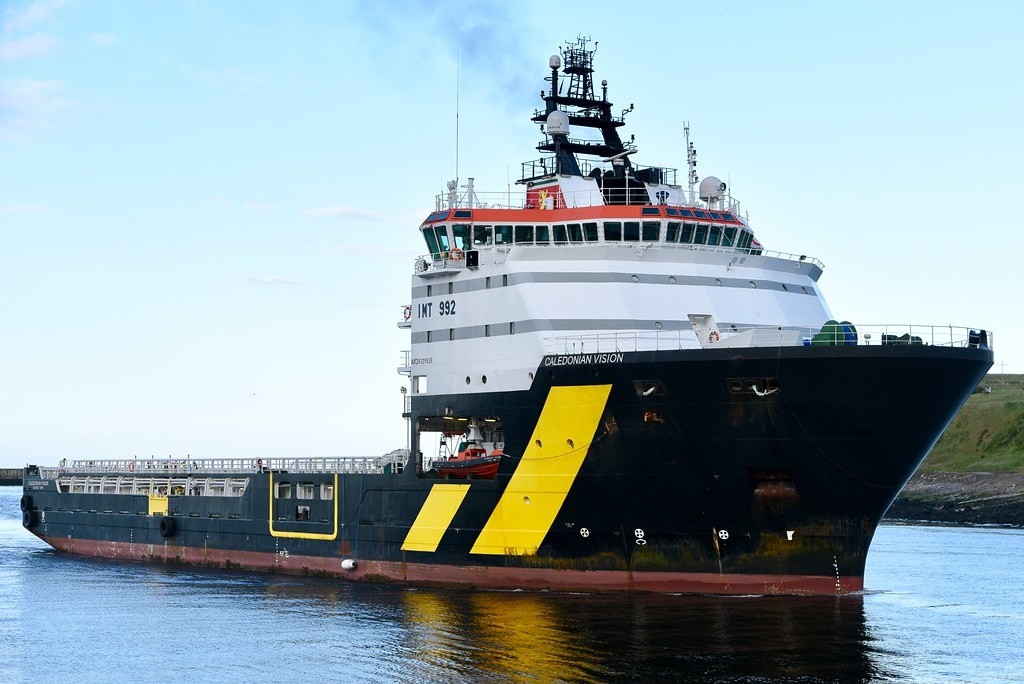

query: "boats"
left=21, top=36, right=996, bottom=594
left=431, top=441, right=503, bottom=478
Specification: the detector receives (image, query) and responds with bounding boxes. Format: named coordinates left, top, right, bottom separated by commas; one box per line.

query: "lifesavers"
left=20, top=495, right=34, bottom=528
left=404, top=307, right=411, bottom=319
left=709, top=331, right=719, bottom=343
left=451, top=249, right=462, bottom=260
left=160, top=517, right=174, bottom=536
